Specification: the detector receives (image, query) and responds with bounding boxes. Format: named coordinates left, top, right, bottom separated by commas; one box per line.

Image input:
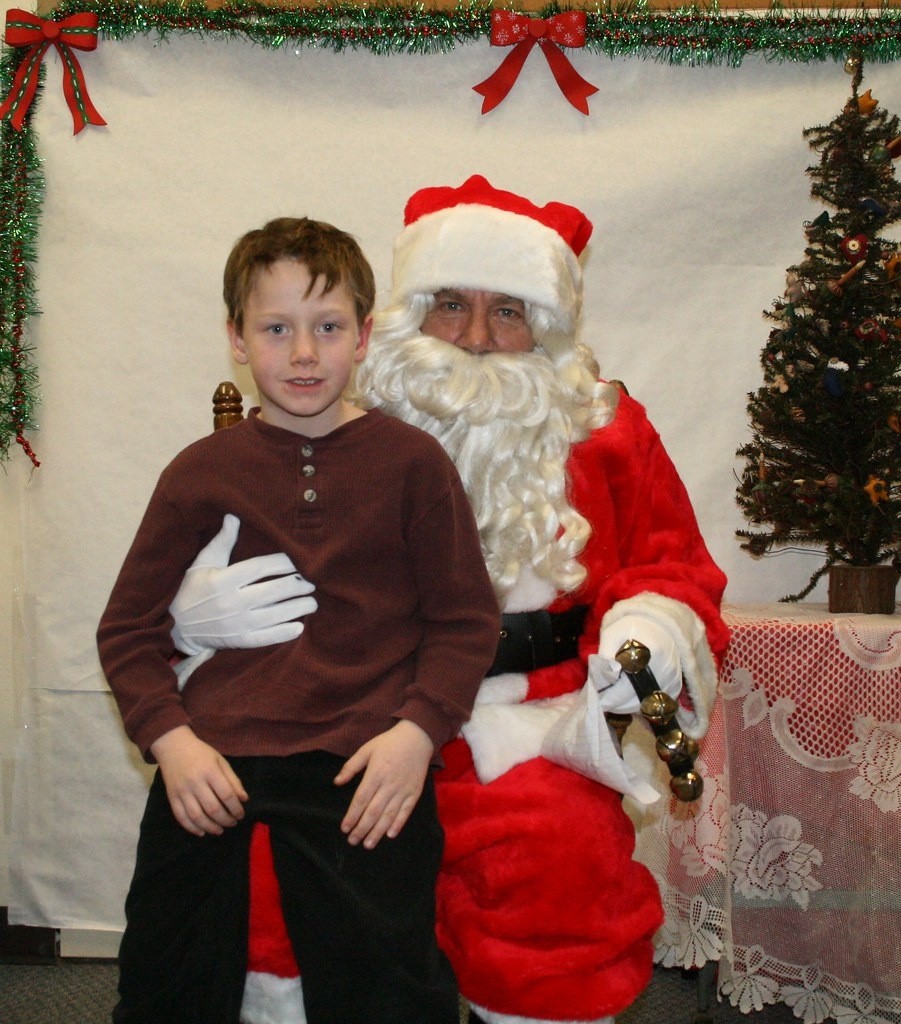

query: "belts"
left=480, top=602, right=592, bottom=680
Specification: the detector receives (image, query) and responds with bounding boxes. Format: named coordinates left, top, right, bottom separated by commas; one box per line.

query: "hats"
left=389, top=175, right=593, bottom=336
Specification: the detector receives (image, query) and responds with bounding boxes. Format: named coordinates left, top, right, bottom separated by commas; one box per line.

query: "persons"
left=95, top=216, right=502, bottom=1024
left=166, top=173, right=732, bottom=1023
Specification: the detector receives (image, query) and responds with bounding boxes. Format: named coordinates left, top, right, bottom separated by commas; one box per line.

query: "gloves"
left=597, top=614, right=682, bottom=717
left=168, top=514, right=318, bottom=657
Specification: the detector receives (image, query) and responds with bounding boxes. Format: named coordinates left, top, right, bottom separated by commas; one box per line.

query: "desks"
left=628, top=605, right=901, bottom=1024
